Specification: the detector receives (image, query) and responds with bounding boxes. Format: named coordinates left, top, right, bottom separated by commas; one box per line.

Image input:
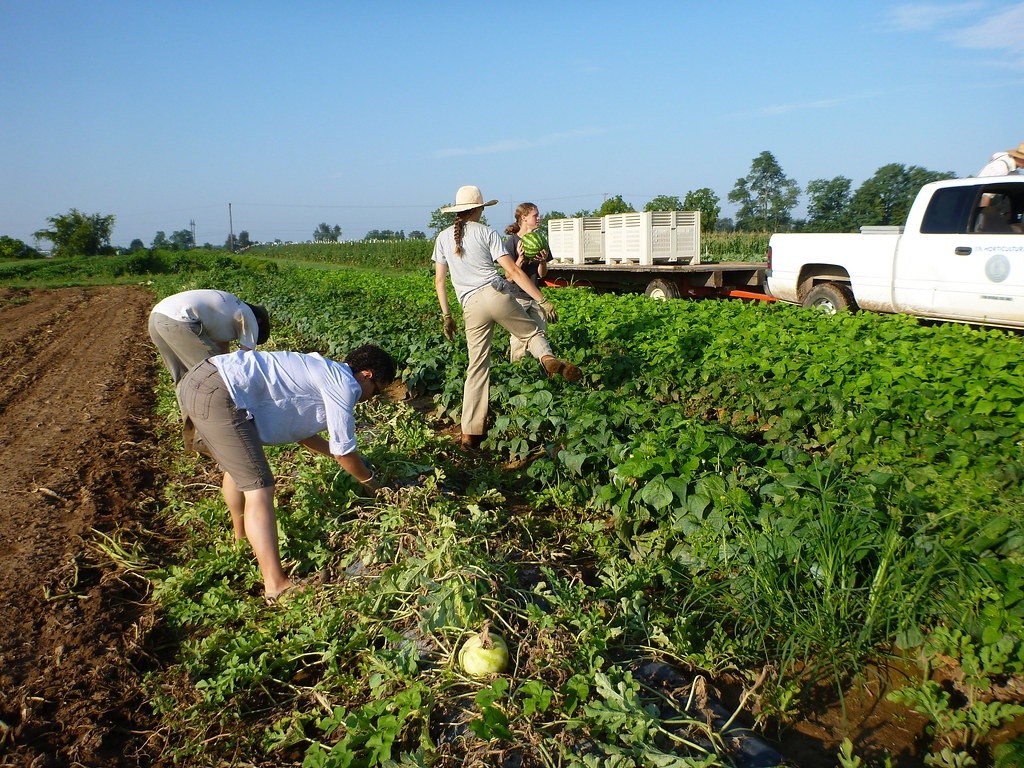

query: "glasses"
left=371, top=377, right=381, bottom=396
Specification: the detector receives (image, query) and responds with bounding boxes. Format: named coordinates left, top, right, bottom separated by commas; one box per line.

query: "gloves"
left=443, top=313, right=458, bottom=343
left=537, top=297, right=559, bottom=324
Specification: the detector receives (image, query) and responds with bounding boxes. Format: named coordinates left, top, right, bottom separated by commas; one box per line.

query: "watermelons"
left=520, top=232, right=548, bottom=258
left=459, top=631, right=508, bottom=677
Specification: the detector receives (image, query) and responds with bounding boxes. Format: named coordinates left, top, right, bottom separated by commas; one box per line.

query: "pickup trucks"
left=764, top=174, right=1024, bottom=330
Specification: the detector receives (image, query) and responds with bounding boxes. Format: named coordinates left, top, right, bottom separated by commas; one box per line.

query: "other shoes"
left=542, top=357, right=583, bottom=381
left=461, top=437, right=475, bottom=448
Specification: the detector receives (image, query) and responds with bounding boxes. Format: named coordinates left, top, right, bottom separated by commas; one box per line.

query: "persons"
left=180, top=344, right=394, bottom=606
left=503, top=203, right=553, bottom=363
left=148, top=289, right=271, bottom=459
left=975, top=142, right=1024, bottom=231
left=431, top=185, right=582, bottom=452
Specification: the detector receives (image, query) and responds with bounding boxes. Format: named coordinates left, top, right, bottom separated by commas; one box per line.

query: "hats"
left=244, top=301, right=271, bottom=345
left=1008, top=144, right=1024, bottom=159
left=442, top=186, right=499, bottom=212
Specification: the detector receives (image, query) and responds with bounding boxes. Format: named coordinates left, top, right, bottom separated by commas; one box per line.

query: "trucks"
left=544, top=257, right=770, bottom=297
